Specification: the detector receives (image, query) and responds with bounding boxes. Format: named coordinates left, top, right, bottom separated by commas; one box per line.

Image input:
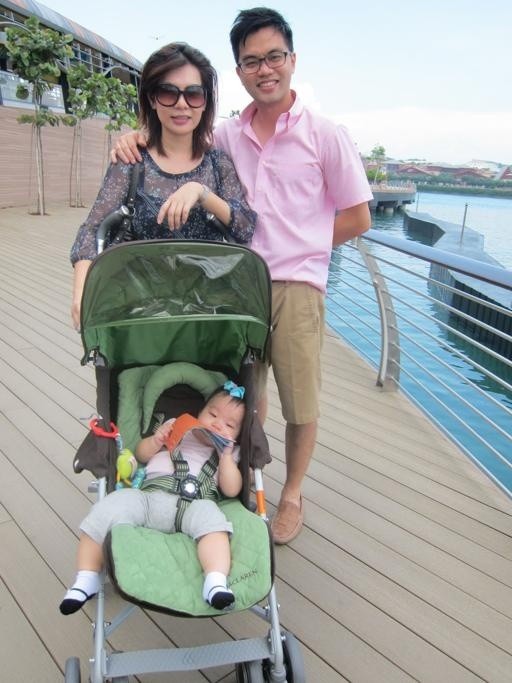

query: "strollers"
left=65, top=206, right=304, bottom=682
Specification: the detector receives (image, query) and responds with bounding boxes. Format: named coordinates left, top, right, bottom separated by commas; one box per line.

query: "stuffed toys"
left=89, top=417, right=147, bottom=491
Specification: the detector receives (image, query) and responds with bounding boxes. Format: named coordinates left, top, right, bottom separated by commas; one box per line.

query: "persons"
left=107, top=7, right=377, bottom=546
left=68, top=39, right=257, bottom=342
left=60, top=380, right=249, bottom=616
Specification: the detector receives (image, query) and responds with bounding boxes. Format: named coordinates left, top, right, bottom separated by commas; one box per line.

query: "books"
left=162, top=411, right=237, bottom=460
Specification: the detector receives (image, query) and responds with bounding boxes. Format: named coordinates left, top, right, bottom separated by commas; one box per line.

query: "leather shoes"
left=269, top=490, right=304, bottom=545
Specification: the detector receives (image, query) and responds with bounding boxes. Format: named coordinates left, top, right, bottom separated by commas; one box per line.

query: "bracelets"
left=197, top=185, right=209, bottom=202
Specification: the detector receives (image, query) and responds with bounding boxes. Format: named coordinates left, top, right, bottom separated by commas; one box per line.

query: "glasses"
left=238, top=51, right=288, bottom=74
left=154, top=83, right=206, bottom=108
